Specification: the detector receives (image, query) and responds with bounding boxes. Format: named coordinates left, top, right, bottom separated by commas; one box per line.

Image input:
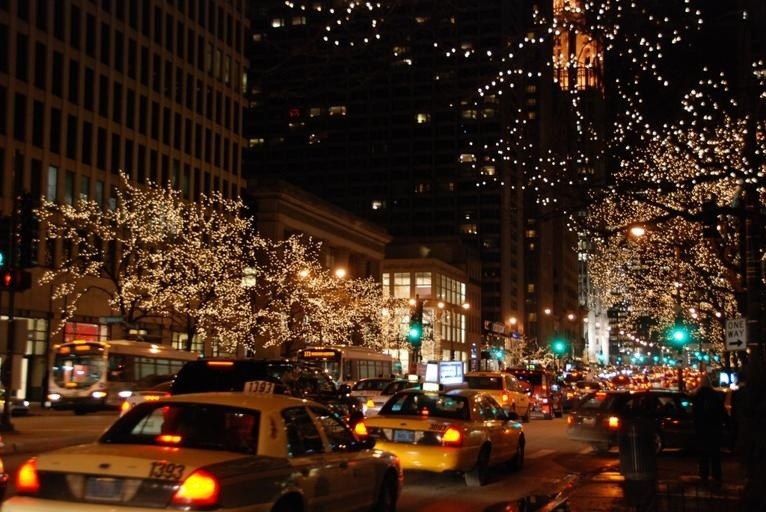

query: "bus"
left=296, top=346, right=392, bottom=382
left=49, top=340, right=203, bottom=414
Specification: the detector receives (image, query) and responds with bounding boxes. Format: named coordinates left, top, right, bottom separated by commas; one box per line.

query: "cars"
left=569, top=390, right=731, bottom=455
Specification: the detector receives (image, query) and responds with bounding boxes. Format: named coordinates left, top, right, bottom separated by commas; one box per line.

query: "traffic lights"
left=0, top=252, right=14, bottom=288
left=408, top=300, right=422, bottom=342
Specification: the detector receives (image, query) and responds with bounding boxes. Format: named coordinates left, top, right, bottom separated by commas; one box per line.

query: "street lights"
left=632, top=226, right=689, bottom=389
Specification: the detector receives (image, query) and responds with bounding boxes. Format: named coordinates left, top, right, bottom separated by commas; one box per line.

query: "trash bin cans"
left=618, top=415, right=656, bottom=482
